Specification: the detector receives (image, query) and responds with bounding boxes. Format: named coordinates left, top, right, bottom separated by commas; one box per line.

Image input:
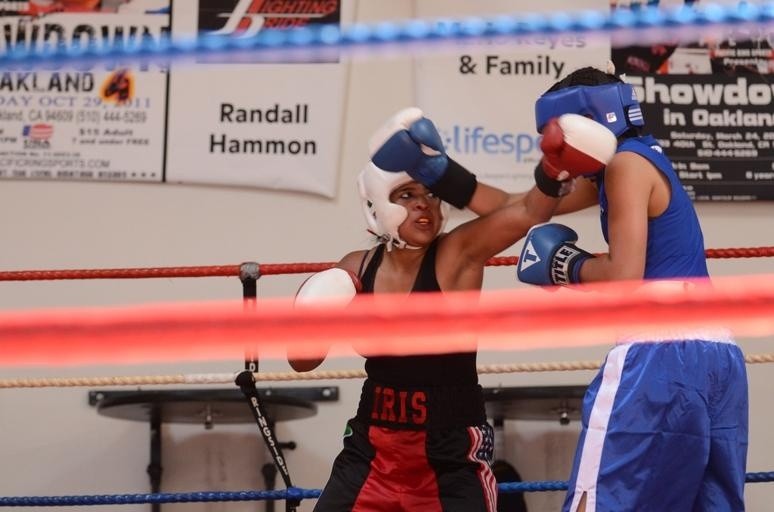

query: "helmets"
left=535, top=82, right=645, bottom=136
left=357, top=161, right=449, bottom=249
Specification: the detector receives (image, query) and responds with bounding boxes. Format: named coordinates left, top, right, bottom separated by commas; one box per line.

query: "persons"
left=367, top=66, right=749, bottom=512
left=284, top=107, right=619, bottom=512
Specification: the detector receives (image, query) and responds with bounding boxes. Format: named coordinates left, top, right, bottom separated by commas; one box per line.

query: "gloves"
left=517, top=222, right=595, bottom=289
left=295, top=266, right=362, bottom=307
left=535, top=113, right=617, bottom=198
left=370, top=107, right=477, bottom=209
